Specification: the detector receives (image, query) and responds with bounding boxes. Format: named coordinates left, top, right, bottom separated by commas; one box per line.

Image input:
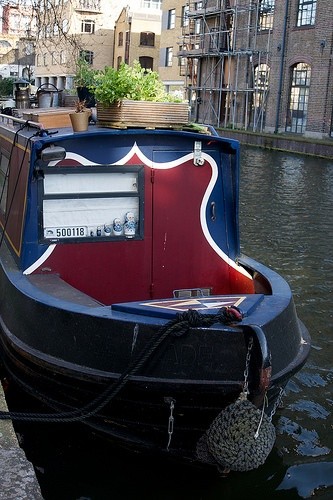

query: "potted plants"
left=72, top=56, right=96, bottom=108
left=68, top=97, right=89, bottom=132
left=93, top=54, right=188, bottom=131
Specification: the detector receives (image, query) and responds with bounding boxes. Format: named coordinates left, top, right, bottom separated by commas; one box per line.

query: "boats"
left=1, top=61, right=313, bottom=469
